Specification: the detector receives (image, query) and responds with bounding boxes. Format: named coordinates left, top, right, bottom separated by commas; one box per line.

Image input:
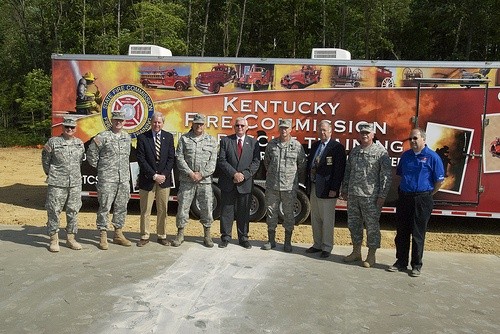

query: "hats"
left=192, top=114, right=207, bottom=124
left=278, top=119, right=292, bottom=128
left=359, top=123, right=374, bottom=133
left=110, top=110, right=126, bottom=121
left=62, top=117, right=77, bottom=127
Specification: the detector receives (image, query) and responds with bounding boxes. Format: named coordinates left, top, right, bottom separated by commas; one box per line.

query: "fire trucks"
left=51, top=45, right=500, bottom=219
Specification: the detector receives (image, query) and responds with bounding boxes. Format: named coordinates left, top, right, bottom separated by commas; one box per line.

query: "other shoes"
left=157, top=239, right=171, bottom=246
left=306, top=247, right=330, bottom=258
left=218, top=240, right=229, bottom=248
left=386, top=261, right=408, bottom=272
left=411, top=266, right=421, bottom=277
left=136, top=239, right=149, bottom=247
left=239, top=240, right=252, bottom=249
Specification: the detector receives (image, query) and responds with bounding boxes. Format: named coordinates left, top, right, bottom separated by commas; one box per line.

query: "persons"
left=304, top=120, right=346, bottom=258
left=385, top=126, right=446, bottom=277
left=134, top=113, right=175, bottom=248
left=218, top=116, right=261, bottom=249
left=171, top=114, right=218, bottom=247
left=84, top=111, right=132, bottom=249
left=342, top=123, right=392, bottom=266
left=262, top=120, right=305, bottom=252
left=40, top=114, right=86, bottom=252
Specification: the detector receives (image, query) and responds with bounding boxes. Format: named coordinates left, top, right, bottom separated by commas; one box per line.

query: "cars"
left=193, top=64, right=236, bottom=95
left=280, top=65, right=321, bottom=89
left=238, top=67, right=273, bottom=88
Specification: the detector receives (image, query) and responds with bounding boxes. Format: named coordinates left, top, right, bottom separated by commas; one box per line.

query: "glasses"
left=409, top=137, right=418, bottom=141
left=64, top=126, right=75, bottom=129
left=234, top=124, right=246, bottom=128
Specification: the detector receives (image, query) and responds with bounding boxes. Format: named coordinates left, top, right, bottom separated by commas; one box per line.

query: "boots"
left=98, top=230, right=109, bottom=250
left=361, top=249, right=376, bottom=268
left=203, top=226, right=214, bottom=248
left=262, top=230, right=276, bottom=250
left=343, top=245, right=362, bottom=262
left=49, top=233, right=60, bottom=253
left=283, top=229, right=293, bottom=252
left=171, top=228, right=185, bottom=247
left=66, top=233, right=83, bottom=250
left=112, top=228, right=132, bottom=246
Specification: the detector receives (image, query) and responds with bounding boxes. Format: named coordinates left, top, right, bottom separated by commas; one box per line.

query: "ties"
left=237, top=138, right=243, bottom=160
left=155, top=133, right=161, bottom=165
left=312, top=143, right=323, bottom=178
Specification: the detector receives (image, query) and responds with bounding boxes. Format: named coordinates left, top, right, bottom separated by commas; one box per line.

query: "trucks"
left=138, top=69, right=190, bottom=91
